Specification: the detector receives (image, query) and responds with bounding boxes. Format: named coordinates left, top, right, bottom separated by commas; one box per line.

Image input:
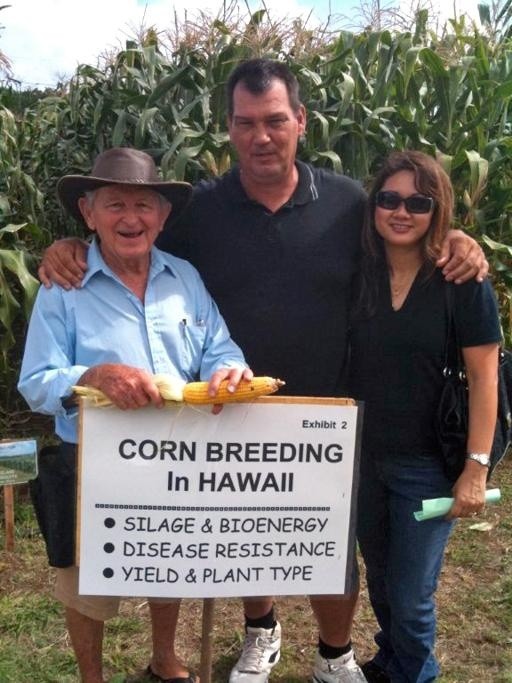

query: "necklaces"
left=386, top=257, right=425, bottom=296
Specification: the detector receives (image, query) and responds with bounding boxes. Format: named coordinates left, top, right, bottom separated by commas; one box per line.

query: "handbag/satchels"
left=437, top=277, right=510, bottom=484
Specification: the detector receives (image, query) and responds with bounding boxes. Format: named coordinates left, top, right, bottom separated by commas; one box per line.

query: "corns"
left=184, top=376, right=286, bottom=404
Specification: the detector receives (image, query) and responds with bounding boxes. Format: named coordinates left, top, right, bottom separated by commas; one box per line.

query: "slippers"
left=147, top=660, right=197, bottom=682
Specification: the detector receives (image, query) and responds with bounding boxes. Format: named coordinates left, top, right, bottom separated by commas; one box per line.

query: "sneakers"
left=311, top=644, right=366, bottom=683
left=362, top=660, right=390, bottom=681
left=228, top=618, right=282, bottom=683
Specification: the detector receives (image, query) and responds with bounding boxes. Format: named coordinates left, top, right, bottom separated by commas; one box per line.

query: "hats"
left=56, top=147, right=192, bottom=221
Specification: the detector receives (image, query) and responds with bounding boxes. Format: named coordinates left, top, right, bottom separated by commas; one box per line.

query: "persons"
left=36, top=57, right=489, bottom=682
left=18, top=146, right=254, bottom=683
left=347, top=147, right=502, bottom=682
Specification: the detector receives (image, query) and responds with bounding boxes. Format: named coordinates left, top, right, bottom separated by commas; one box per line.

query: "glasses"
left=375, top=190, right=433, bottom=214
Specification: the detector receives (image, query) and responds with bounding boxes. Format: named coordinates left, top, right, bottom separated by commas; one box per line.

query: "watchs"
left=464, top=451, right=492, bottom=468
